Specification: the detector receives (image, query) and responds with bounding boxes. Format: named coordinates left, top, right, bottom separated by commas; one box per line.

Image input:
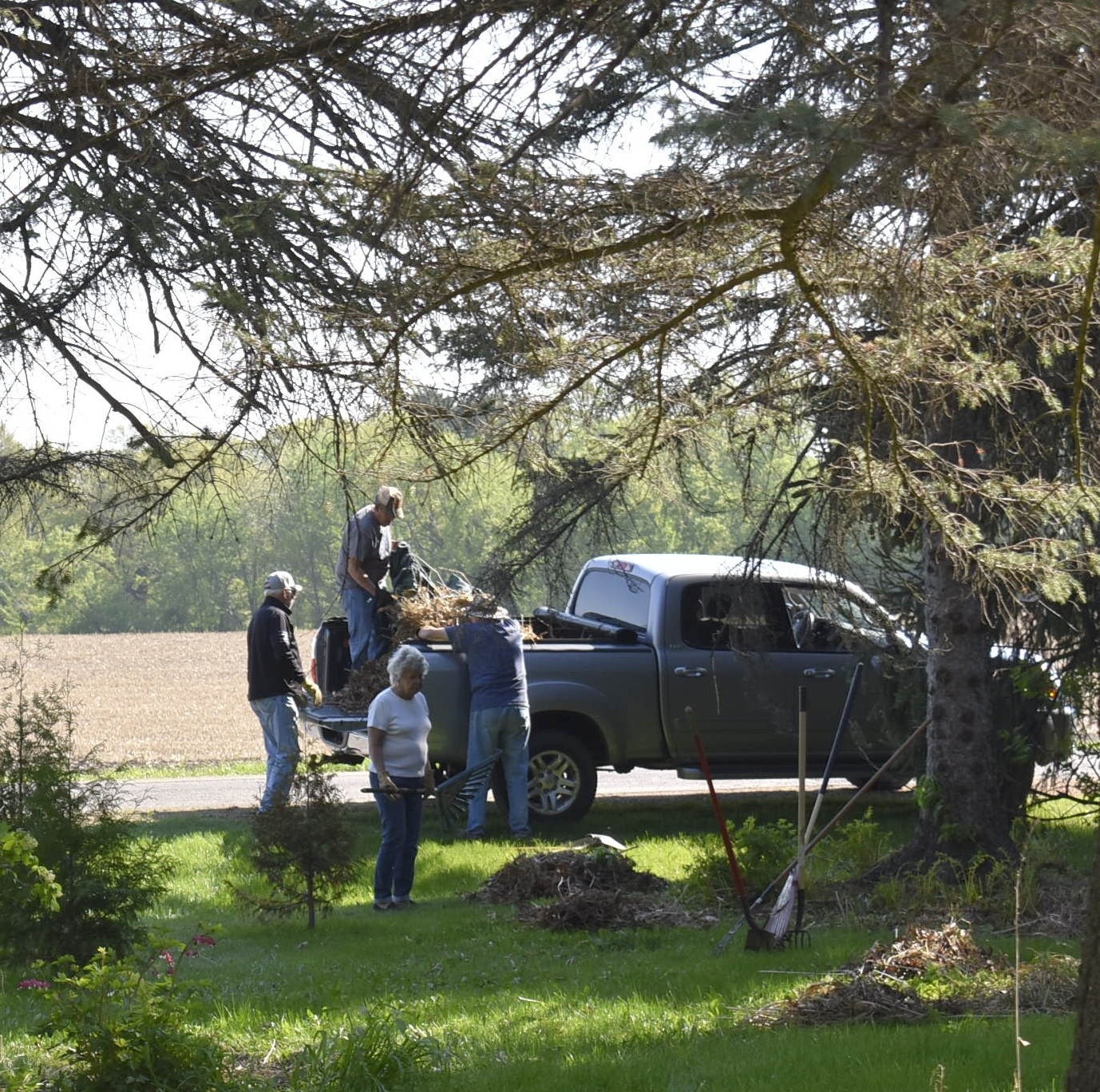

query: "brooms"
left=759, top=661, right=865, bottom=943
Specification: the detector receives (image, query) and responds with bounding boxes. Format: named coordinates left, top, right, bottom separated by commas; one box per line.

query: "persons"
left=247, top=571, right=323, bottom=810
left=418, top=593, right=532, bottom=840
left=367, top=645, right=435, bottom=911
left=335, top=485, right=405, bottom=670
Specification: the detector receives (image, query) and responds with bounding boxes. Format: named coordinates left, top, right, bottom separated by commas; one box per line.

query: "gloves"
left=301, top=678, right=323, bottom=705
left=374, top=588, right=393, bottom=604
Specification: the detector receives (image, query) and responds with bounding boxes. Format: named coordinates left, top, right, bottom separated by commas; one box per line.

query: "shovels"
left=765, top=661, right=865, bottom=937
left=691, top=733, right=777, bottom=955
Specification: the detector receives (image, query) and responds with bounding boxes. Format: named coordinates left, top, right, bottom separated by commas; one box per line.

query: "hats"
left=375, top=486, right=404, bottom=519
left=263, top=571, right=302, bottom=592
left=460, top=594, right=509, bottom=619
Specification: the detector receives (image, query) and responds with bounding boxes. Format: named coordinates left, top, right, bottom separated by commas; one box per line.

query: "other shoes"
left=393, top=899, right=417, bottom=908
left=373, top=901, right=403, bottom=912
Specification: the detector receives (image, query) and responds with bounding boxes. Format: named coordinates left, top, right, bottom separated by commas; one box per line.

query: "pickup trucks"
left=283, top=552, right=1077, bottom=825
